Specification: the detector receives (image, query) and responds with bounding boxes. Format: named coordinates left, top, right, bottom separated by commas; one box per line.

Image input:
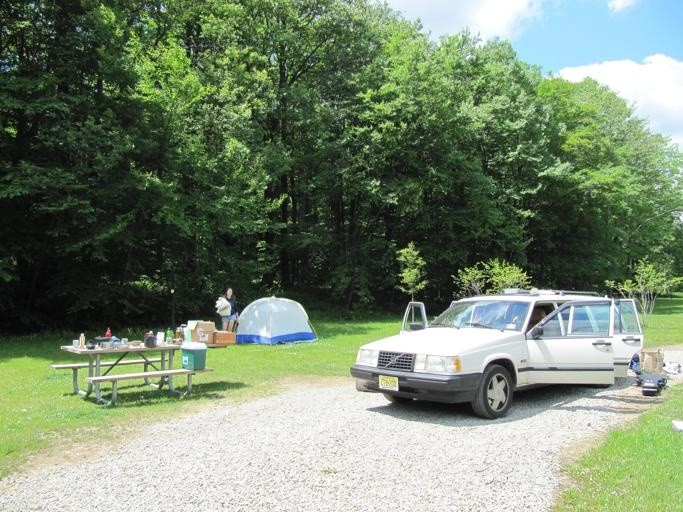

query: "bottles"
left=144, top=323, right=191, bottom=348
left=105, top=329, right=112, bottom=338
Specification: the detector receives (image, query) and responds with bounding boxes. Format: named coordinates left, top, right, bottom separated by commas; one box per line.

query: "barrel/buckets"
left=164, top=326, right=172, bottom=337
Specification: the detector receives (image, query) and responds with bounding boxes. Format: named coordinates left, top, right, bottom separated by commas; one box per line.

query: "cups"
left=120, top=337, right=128, bottom=346
left=73, top=339, right=79, bottom=349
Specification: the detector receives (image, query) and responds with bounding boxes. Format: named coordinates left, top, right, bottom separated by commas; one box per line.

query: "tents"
left=234, top=294, right=319, bottom=346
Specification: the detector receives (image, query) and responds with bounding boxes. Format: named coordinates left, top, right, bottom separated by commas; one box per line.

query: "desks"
left=60, top=344, right=180, bottom=403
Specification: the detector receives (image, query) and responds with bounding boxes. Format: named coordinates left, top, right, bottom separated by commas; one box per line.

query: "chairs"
left=525, top=308, right=547, bottom=335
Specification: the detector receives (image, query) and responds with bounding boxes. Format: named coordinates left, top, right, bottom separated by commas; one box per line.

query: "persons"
left=219, top=286, right=238, bottom=332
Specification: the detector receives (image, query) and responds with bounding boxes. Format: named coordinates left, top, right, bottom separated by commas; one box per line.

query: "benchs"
left=50, top=359, right=169, bottom=397
left=85, top=367, right=215, bottom=405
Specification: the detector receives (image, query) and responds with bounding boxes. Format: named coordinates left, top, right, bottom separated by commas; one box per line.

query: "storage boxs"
left=214, top=331, right=236, bottom=344
left=638, top=351, right=665, bottom=372
left=187, top=320, right=216, bottom=343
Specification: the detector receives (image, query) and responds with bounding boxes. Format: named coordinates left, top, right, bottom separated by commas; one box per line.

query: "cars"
left=349, top=285, right=651, bottom=419
